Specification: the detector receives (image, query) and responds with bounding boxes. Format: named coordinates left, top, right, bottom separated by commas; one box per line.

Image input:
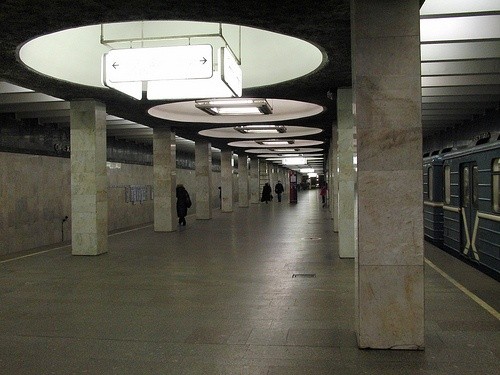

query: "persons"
left=263, top=183, right=272, bottom=204
left=275, top=180, right=284, bottom=202
left=301, top=180, right=307, bottom=190
left=176, top=184, right=191, bottom=226
left=320, top=182, right=327, bottom=203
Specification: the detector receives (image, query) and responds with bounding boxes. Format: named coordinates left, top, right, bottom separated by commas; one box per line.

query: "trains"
left=422, top=133, right=499, bottom=273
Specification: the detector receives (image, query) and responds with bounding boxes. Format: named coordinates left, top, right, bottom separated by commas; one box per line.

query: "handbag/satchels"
left=186, top=198, right=192, bottom=208
left=261, top=197, right=264, bottom=202
